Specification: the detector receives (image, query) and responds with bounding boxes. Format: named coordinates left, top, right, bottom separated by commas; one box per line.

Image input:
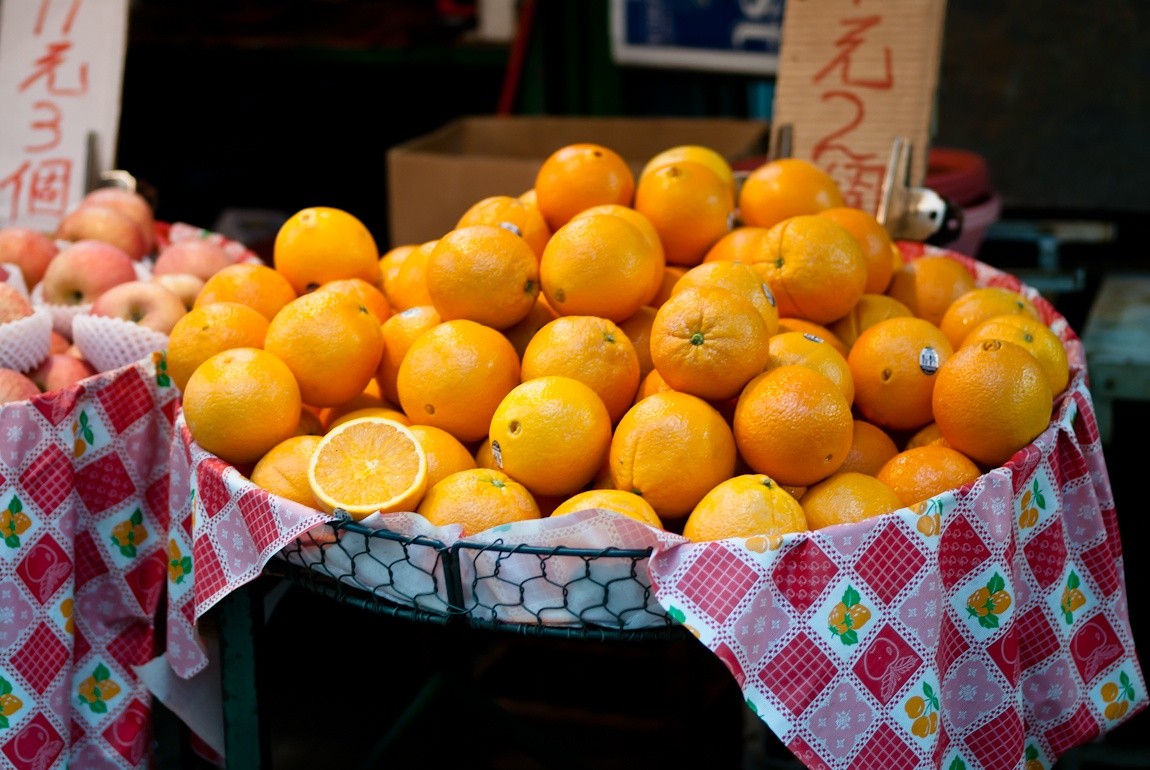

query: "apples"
left=2, top=186, right=231, bottom=405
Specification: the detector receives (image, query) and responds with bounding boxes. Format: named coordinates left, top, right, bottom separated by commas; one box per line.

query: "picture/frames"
left=609, top=0, right=785, bottom=79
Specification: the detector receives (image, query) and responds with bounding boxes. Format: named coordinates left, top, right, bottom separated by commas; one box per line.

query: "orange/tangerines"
left=168, top=143, right=1072, bottom=545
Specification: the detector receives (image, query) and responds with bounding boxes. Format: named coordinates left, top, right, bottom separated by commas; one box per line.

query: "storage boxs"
left=385, top=109, right=766, bottom=262
left=0, top=218, right=260, bottom=770
